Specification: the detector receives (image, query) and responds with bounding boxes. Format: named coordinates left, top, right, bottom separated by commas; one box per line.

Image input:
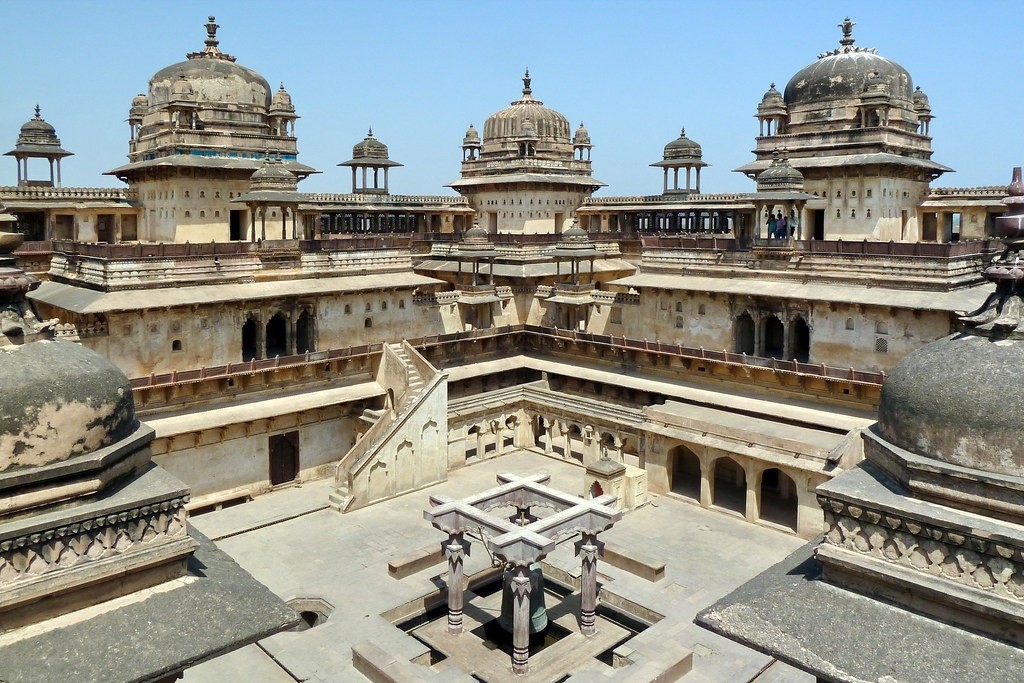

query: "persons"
left=766, top=210, right=798, bottom=241
